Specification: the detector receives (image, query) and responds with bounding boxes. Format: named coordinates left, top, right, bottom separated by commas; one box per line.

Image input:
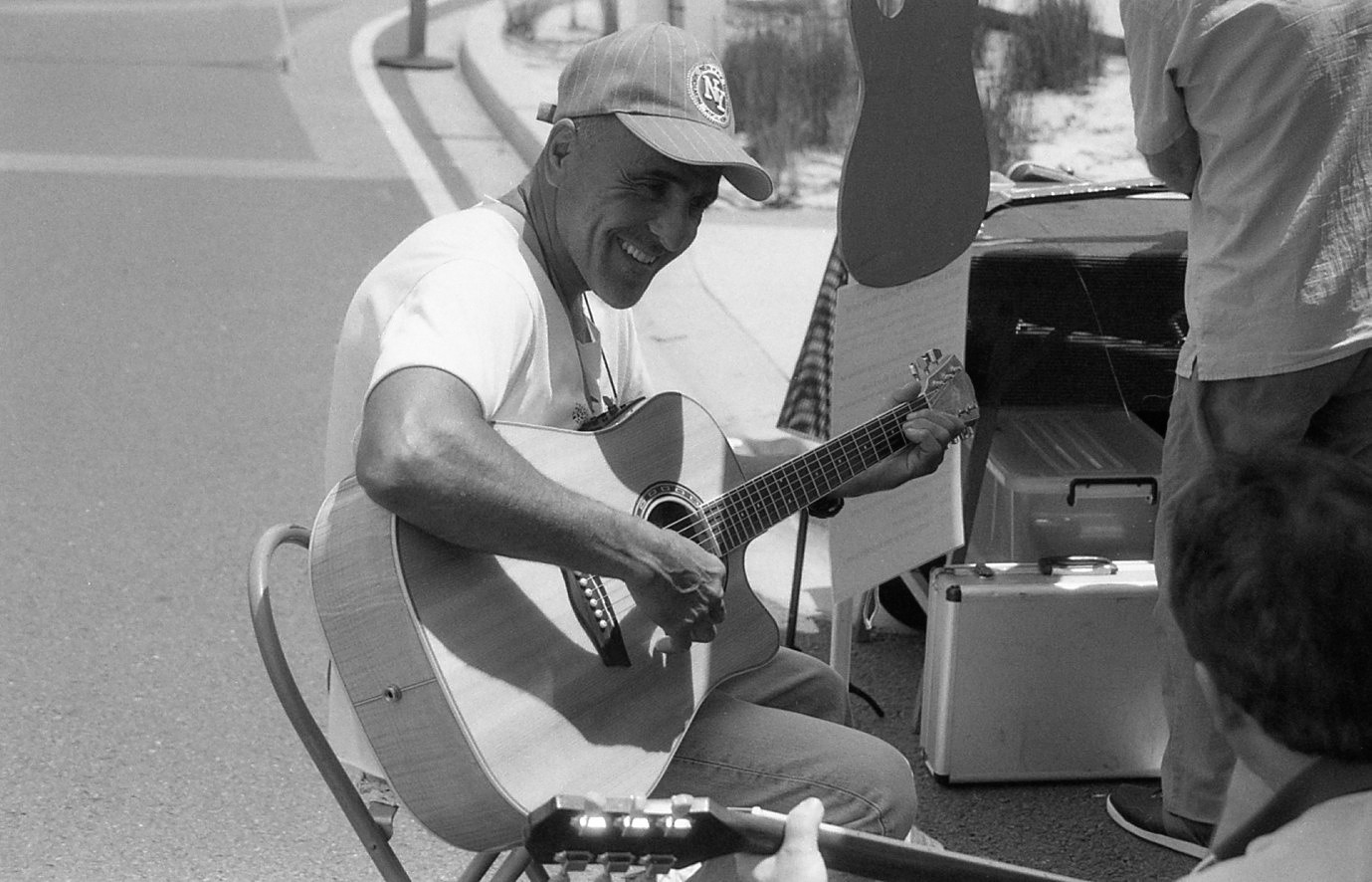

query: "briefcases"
left=920, top=553, right=1168, bottom=788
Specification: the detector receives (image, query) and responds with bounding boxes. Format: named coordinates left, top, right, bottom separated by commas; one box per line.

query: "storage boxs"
left=958, top=403, right=1168, bottom=565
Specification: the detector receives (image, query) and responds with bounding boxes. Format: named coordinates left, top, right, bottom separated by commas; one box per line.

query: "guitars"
left=307, top=348, right=981, bottom=854
left=835, top=0, right=992, bottom=289
left=521, top=792, right=1104, bottom=882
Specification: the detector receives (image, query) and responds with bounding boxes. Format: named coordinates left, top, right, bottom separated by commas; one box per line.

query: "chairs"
left=246, top=522, right=768, bottom=881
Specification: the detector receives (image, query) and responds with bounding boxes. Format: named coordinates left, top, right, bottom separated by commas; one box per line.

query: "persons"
left=325, top=20, right=962, bottom=852
left=751, top=438, right=1372, bottom=881
left=1105, top=1, right=1372, bottom=860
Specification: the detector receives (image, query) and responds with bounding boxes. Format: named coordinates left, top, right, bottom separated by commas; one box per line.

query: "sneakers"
left=1105, top=782, right=1220, bottom=860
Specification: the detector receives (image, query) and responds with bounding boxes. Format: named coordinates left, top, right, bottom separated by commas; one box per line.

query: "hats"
left=536, top=22, right=776, bottom=202
